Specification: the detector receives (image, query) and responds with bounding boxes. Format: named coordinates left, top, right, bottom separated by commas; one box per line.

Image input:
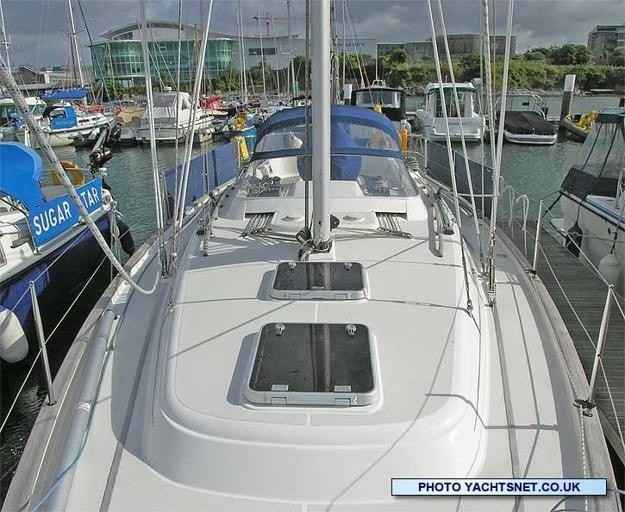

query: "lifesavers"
left=51, top=162, right=83, bottom=186
left=235, top=118, right=243, bottom=126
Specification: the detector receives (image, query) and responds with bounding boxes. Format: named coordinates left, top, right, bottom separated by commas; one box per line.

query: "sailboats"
left=1, top=0, right=625, bottom=512
